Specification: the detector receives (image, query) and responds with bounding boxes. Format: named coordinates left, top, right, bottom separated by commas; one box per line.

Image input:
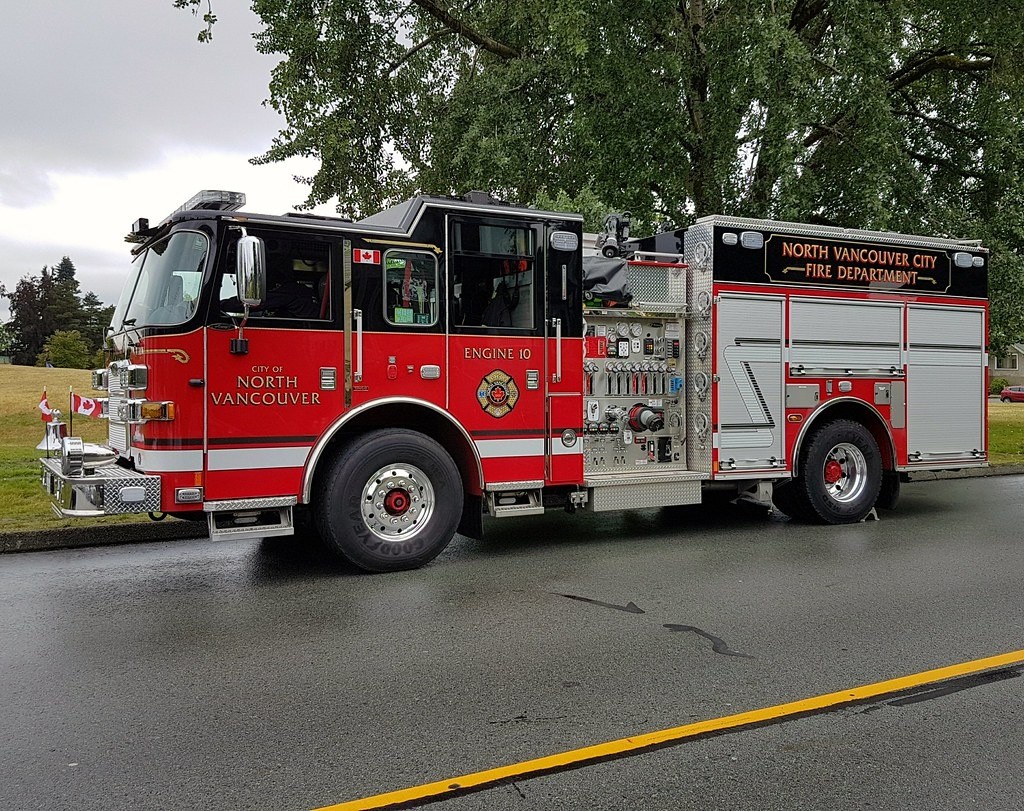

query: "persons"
left=219, top=253, right=316, bottom=319
left=46, top=354, right=52, bottom=368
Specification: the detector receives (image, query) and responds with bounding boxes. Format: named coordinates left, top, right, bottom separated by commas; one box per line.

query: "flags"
left=38, top=391, right=53, bottom=422
left=70, top=392, right=101, bottom=417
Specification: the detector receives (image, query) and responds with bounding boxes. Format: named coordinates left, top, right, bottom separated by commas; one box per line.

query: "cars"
left=1000, top=385, right=1024, bottom=403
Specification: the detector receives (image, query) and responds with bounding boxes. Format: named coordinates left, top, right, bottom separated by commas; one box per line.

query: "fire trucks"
left=37, top=189, right=990, bottom=574
